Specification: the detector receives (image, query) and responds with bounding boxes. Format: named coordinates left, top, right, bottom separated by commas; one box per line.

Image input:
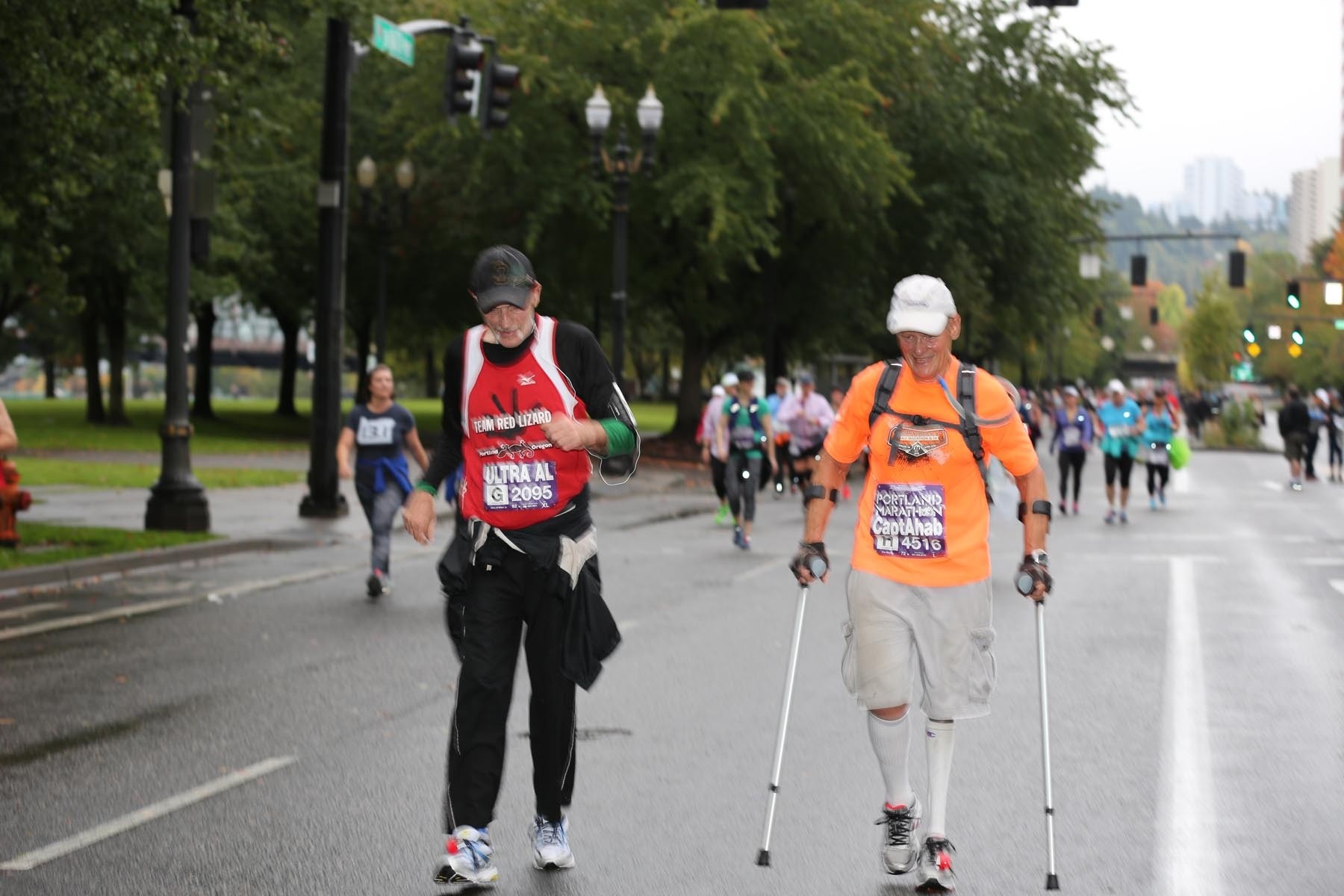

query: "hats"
left=469, top=245, right=536, bottom=314
left=1062, top=385, right=1079, bottom=397
left=1106, top=378, right=1126, bottom=396
left=711, top=383, right=724, bottom=397
left=721, top=372, right=738, bottom=388
left=886, top=273, right=958, bottom=337
left=799, top=372, right=813, bottom=383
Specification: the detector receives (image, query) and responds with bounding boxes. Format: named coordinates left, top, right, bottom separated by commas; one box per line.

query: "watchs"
left=1024, top=549, right=1049, bottom=567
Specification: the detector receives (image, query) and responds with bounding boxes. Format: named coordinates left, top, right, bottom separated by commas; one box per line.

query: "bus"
left=1121, top=355, right=1181, bottom=401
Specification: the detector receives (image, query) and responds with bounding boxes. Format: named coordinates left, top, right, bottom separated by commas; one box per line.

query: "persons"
left=336, top=362, right=433, bottom=596
left=400, top=247, right=638, bottom=884
left=0, top=399, right=19, bottom=461
left=788, top=274, right=1058, bottom=893
left=698, top=363, right=1344, bottom=553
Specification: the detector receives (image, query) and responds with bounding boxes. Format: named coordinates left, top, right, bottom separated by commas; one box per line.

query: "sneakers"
left=874, top=791, right=923, bottom=875
left=914, top=834, right=959, bottom=893
left=430, top=827, right=500, bottom=885
left=528, top=812, right=579, bottom=871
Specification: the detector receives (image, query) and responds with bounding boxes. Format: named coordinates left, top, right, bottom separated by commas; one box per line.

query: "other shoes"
left=1103, top=508, right=1116, bottom=525
left=1118, top=511, right=1128, bottom=522
left=365, top=570, right=394, bottom=595
left=1149, top=491, right=1168, bottom=511
left=1059, top=500, right=1067, bottom=514
left=733, top=526, right=752, bottom=550
left=714, top=505, right=731, bottom=522
left=1072, top=501, right=1079, bottom=514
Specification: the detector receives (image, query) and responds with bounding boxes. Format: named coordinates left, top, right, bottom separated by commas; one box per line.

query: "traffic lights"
left=1241, top=323, right=1255, bottom=345
left=441, top=33, right=486, bottom=117
left=1292, top=324, right=1304, bottom=348
left=1284, top=281, right=1303, bottom=312
left=481, top=55, right=521, bottom=130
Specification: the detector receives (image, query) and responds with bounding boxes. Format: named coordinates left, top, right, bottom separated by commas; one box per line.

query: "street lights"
left=355, top=154, right=418, bottom=392
left=578, top=80, right=667, bottom=397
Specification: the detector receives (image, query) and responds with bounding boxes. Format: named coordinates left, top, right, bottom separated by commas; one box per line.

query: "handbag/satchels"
left=1165, top=434, right=1192, bottom=469
